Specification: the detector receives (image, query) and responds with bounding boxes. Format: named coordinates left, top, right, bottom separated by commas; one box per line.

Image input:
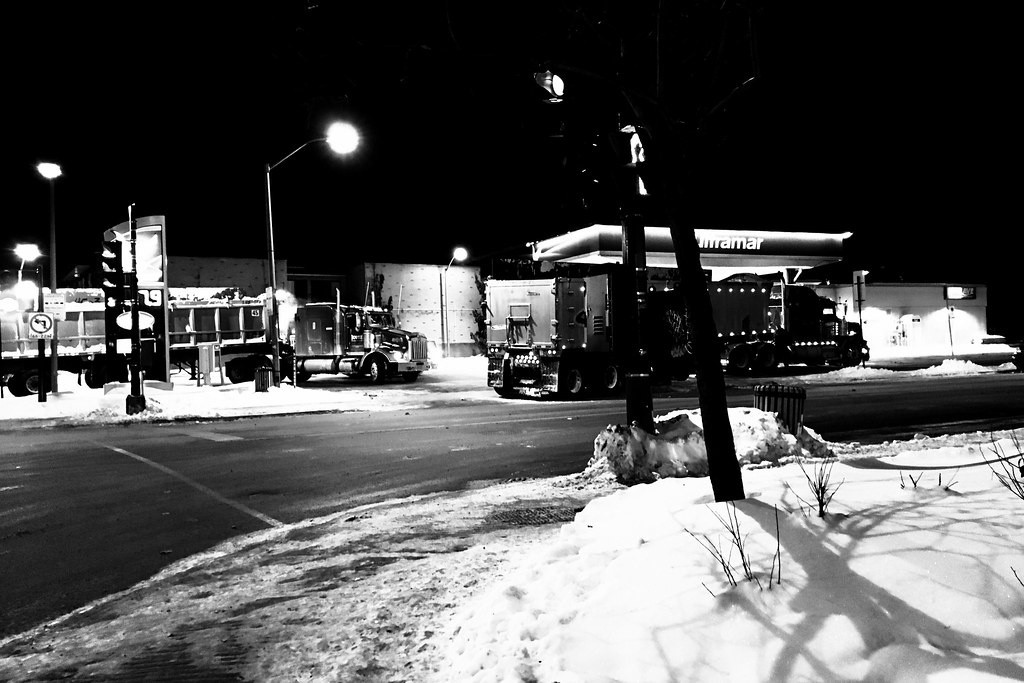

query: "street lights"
left=13, top=242, right=42, bottom=284
left=263, top=122, right=358, bottom=385
left=444, top=246, right=468, bottom=359
left=36, top=160, right=63, bottom=392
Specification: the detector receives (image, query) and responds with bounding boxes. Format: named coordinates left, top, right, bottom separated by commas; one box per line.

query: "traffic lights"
left=98, top=237, right=121, bottom=299
left=522, top=61, right=570, bottom=104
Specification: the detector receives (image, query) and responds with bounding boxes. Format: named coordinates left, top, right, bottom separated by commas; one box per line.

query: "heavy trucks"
left=484, top=265, right=871, bottom=400
left=0, top=285, right=430, bottom=398
